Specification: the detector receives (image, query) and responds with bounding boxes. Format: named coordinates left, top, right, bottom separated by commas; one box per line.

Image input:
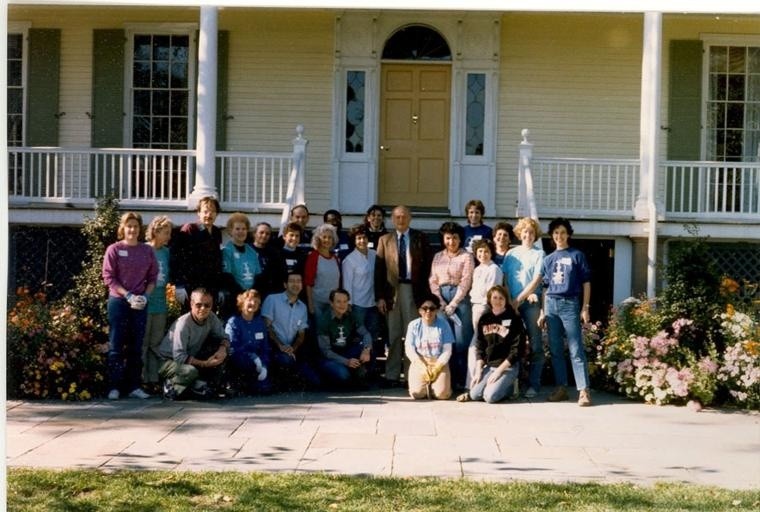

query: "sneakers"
left=108, top=388, right=150, bottom=399
left=525, top=387, right=592, bottom=405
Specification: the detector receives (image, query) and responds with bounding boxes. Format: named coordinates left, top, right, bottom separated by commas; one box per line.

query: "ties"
left=398, top=234, right=406, bottom=280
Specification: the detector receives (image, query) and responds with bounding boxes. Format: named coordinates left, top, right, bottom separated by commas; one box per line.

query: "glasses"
left=422, top=307, right=436, bottom=311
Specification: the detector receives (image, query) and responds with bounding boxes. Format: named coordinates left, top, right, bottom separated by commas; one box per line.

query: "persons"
left=102, top=195, right=597, bottom=407
